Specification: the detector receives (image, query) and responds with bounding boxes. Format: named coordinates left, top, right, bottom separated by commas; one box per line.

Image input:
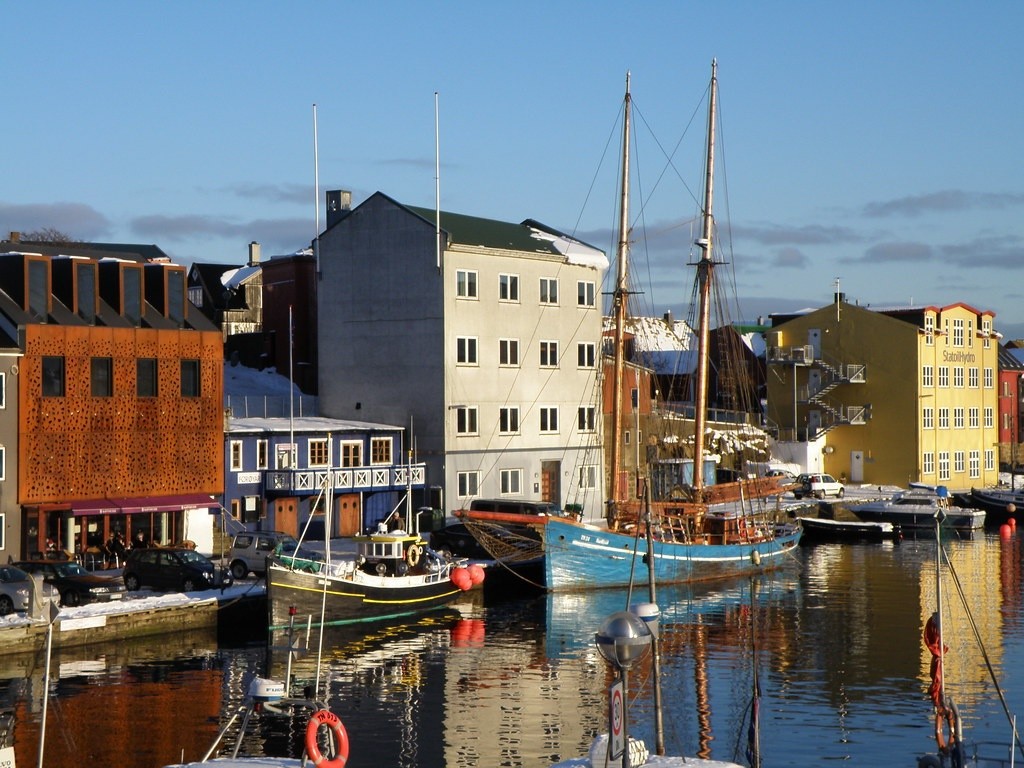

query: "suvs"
left=228, top=530, right=325, bottom=582
left=793, top=472, right=846, bottom=500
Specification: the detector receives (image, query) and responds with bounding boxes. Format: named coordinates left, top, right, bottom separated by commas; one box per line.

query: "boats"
left=788, top=508, right=904, bottom=545
left=841, top=480, right=987, bottom=530
left=970, top=485, right=1024, bottom=522
left=911, top=521, right=1024, bottom=768
left=540, top=477, right=749, bottom=768
left=0, top=620, right=100, bottom=768
left=265, top=419, right=523, bottom=633
left=159, top=607, right=352, bottom=768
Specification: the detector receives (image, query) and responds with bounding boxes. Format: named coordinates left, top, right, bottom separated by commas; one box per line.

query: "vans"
left=468, top=497, right=566, bottom=541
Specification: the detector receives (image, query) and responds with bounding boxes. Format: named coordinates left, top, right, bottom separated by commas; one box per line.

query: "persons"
left=104, top=532, right=150, bottom=568
left=389, top=512, right=406, bottom=532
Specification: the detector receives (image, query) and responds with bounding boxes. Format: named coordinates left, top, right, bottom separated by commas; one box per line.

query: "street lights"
left=593, top=610, right=653, bottom=768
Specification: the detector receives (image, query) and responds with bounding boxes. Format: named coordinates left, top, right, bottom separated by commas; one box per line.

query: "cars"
left=12, top=559, right=128, bottom=607
left=0, top=562, right=62, bottom=617
left=764, top=470, right=798, bottom=487
left=428, top=523, right=539, bottom=561
left=123, top=546, right=235, bottom=593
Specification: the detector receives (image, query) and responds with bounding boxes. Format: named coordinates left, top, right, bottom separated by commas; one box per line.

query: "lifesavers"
left=305, top=711, right=350, bottom=768
left=406, top=544, right=420, bottom=567
left=935, top=707, right=955, bottom=747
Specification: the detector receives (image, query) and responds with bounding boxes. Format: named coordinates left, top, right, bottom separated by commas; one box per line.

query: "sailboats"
left=448, top=56, right=806, bottom=594
